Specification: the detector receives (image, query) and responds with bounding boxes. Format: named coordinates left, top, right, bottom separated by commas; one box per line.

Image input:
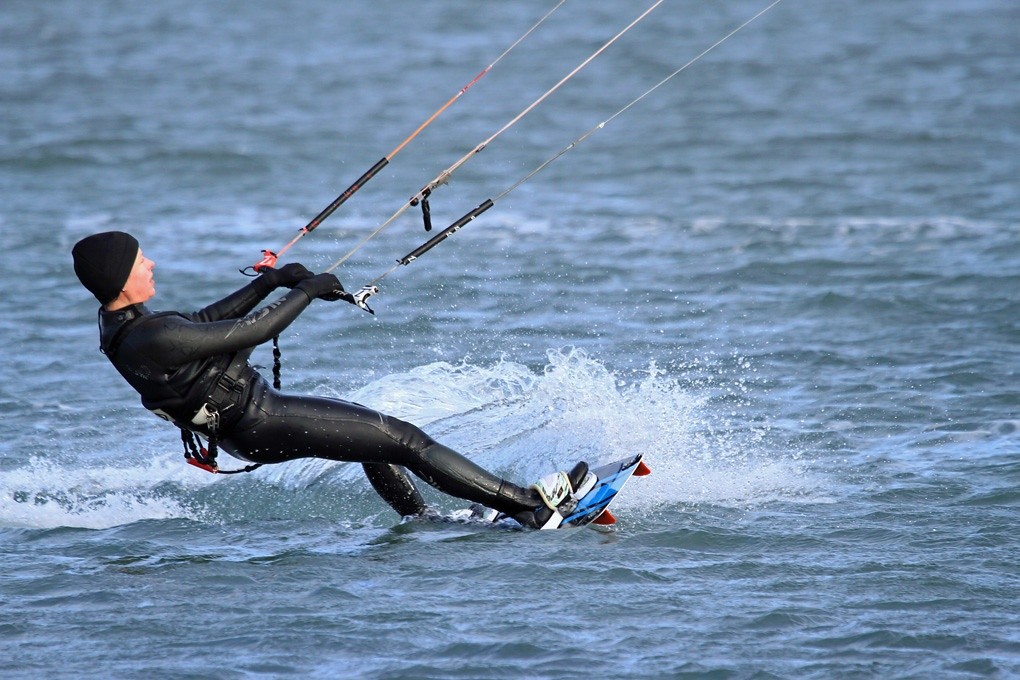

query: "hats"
left=71, top=231, right=139, bottom=306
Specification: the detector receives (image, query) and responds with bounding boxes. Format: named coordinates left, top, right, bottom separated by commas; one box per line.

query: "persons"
left=72, top=231, right=589, bottom=533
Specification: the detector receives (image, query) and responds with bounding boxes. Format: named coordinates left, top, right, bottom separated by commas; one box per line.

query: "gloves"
left=263, top=263, right=307, bottom=291
left=300, top=273, right=344, bottom=304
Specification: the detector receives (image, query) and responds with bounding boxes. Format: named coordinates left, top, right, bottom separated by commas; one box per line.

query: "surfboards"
left=486, top=452, right=651, bottom=528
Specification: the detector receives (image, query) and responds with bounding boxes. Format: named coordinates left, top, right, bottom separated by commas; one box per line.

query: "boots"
left=468, top=504, right=484, bottom=518
left=490, top=461, right=589, bottom=530
